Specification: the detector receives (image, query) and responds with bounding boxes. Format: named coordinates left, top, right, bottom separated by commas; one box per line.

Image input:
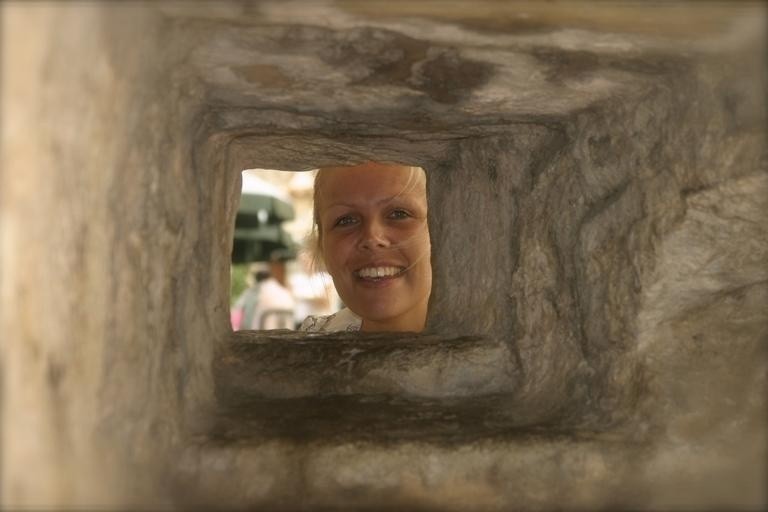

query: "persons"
left=297, top=163, right=432, bottom=333
left=231, top=248, right=342, bottom=331
